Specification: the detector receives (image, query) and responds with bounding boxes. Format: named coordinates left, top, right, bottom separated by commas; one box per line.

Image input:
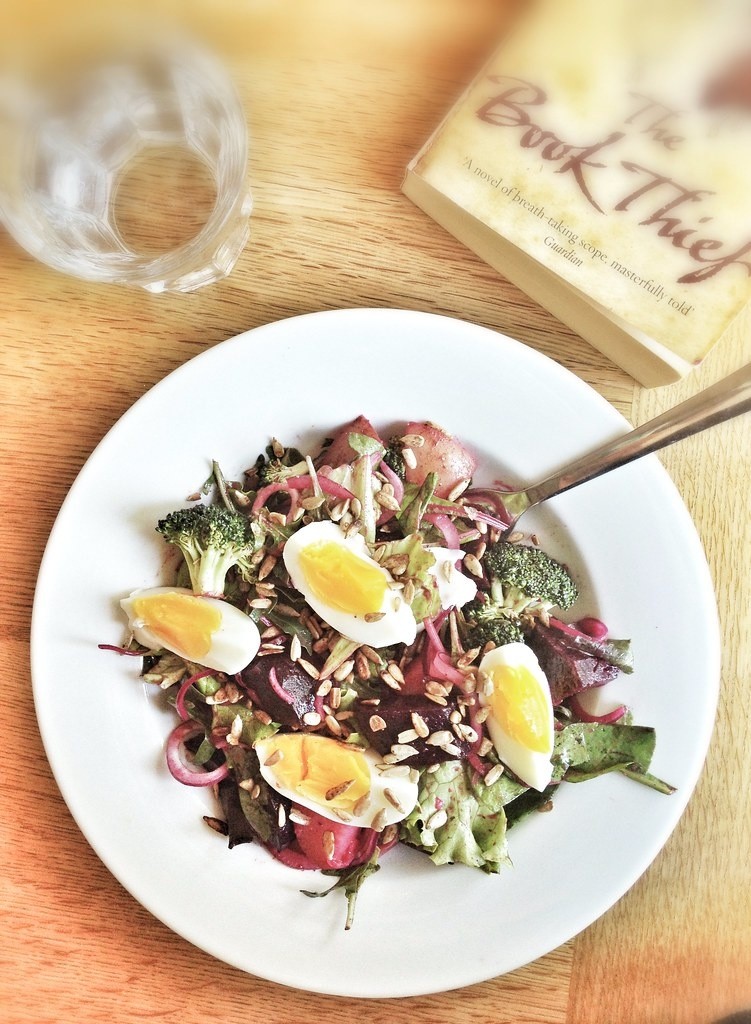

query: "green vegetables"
left=399, top=719, right=677, bottom=874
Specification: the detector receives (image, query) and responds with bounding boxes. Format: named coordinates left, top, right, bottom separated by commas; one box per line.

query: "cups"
left=0, top=20, right=255, bottom=295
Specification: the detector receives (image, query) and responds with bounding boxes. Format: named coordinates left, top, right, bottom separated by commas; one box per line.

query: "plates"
left=30, top=309, right=720, bottom=998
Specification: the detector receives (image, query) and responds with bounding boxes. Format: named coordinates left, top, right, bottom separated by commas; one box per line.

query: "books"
left=390, top=0, right=751, bottom=390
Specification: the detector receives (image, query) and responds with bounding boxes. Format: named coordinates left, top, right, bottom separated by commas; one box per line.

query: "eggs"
left=281, top=521, right=417, bottom=648
left=478, top=642, right=554, bottom=794
left=119, top=587, right=261, bottom=675
left=255, top=733, right=420, bottom=827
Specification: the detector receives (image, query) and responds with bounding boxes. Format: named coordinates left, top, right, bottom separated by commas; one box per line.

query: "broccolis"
left=470, top=543, right=578, bottom=656
left=155, top=503, right=256, bottom=597
left=260, top=459, right=309, bottom=495
